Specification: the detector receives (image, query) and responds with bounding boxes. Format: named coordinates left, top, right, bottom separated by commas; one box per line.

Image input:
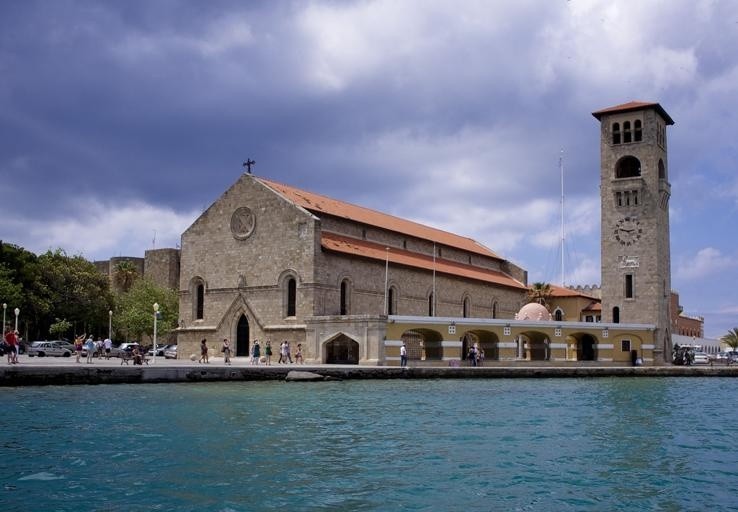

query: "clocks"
left=612, top=215, right=642, bottom=247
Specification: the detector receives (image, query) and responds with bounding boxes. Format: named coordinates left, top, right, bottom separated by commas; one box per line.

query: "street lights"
left=107, top=310, right=113, bottom=340
left=693, top=336, right=696, bottom=352
left=2, top=302, right=8, bottom=339
left=152, top=302, right=160, bottom=364
left=14, top=307, right=22, bottom=331
left=384, top=246, right=391, bottom=316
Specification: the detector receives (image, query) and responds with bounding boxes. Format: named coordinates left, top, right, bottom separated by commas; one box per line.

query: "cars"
left=81, top=342, right=178, bottom=359
left=687, top=351, right=738, bottom=365
left=0, top=339, right=26, bottom=356
left=52, top=340, right=75, bottom=353
left=27, top=341, right=73, bottom=357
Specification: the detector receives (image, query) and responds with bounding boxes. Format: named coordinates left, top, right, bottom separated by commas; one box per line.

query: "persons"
left=6, top=329, right=20, bottom=363
left=85, top=334, right=92, bottom=363
left=198, top=339, right=303, bottom=365
left=104, top=335, right=111, bottom=360
left=131, top=346, right=143, bottom=363
left=95, top=337, right=103, bottom=358
left=75, top=335, right=82, bottom=362
left=467, top=343, right=485, bottom=366
left=400, top=344, right=407, bottom=367
left=685, top=350, right=690, bottom=365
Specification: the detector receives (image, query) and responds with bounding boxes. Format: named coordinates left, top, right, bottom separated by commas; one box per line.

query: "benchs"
left=117, top=350, right=150, bottom=366
left=708, top=356, right=733, bottom=366
